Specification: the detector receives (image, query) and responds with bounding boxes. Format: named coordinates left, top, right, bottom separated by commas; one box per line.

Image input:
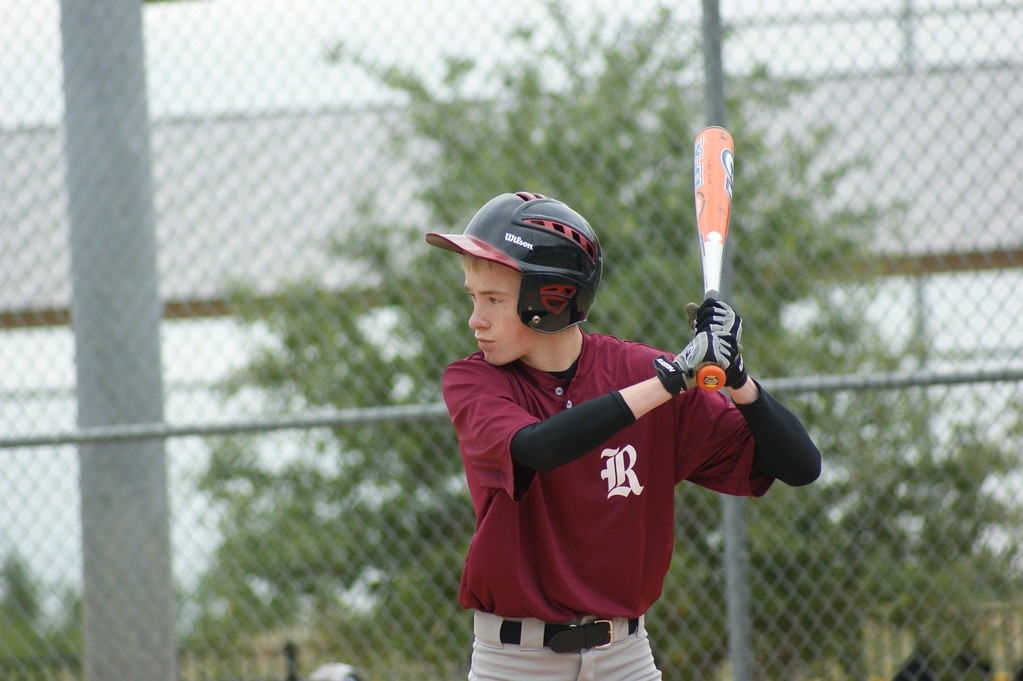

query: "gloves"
left=654, top=299, right=749, bottom=396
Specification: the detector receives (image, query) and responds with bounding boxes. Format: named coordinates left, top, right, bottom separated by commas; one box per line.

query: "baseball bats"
left=694, top=126, right=733, bottom=392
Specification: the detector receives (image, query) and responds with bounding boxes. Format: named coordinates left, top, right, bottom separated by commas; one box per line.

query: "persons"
left=427, top=192, right=821, bottom=681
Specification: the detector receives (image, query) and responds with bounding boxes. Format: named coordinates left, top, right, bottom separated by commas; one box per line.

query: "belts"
left=500, top=618, right=636, bottom=655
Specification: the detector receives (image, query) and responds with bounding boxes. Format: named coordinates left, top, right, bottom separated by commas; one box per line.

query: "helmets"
left=427, top=193, right=605, bottom=334
